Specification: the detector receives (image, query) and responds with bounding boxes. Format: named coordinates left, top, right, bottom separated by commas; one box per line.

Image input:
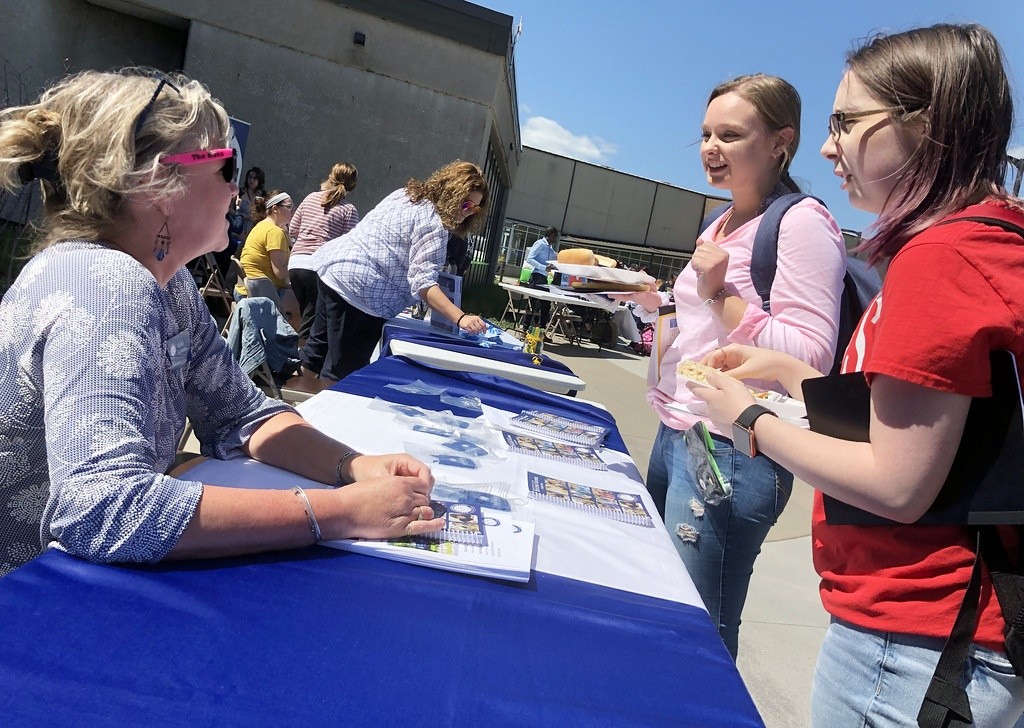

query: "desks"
left=380, top=314, right=586, bottom=397
left=498, top=282, right=605, bottom=347
left=1, top=355, right=766, bottom=728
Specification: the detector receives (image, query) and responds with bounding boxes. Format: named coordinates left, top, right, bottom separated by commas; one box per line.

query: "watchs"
left=733, top=404, right=778, bottom=459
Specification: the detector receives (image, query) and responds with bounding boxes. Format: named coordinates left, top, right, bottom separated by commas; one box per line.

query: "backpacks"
left=699, top=193, right=883, bottom=376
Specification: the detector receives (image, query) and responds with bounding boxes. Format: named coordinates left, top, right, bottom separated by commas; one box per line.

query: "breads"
left=679, top=361, right=745, bottom=390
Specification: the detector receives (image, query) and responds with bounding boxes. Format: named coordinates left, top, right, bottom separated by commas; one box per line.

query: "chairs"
left=177, top=252, right=338, bottom=451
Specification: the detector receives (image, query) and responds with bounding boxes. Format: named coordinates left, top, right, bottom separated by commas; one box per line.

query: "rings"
left=715, top=346, right=727, bottom=361
left=418, top=506, right=424, bottom=520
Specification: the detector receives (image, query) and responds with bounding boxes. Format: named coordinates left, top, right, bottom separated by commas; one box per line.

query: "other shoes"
left=543, top=336, right=553, bottom=343
left=521, top=331, right=526, bottom=338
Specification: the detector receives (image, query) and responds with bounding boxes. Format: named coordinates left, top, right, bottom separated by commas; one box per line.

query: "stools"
left=440, top=271, right=463, bottom=311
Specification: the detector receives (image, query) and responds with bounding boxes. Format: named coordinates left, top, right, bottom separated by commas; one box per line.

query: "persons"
left=608, top=72, right=847, bottom=664
left=520, top=224, right=559, bottom=342
left=686, top=24, right=1024, bottom=728
left=213, top=163, right=359, bottom=346
left=0, top=67, right=447, bottom=578
left=300, top=159, right=490, bottom=382
left=560, top=256, right=678, bottom=333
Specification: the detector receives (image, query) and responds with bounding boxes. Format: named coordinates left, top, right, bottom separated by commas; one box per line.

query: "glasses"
left=828, top=105, right=906, bottom=142
left=279, top=205, right=293, bottom=210
left=462, top=200, right=481, bottom=214
left=135, top=79, right=180, bottom=137
left=159, top=147, right=237, bottom=183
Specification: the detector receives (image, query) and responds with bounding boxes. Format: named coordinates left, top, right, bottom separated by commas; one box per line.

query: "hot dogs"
left=557, top=248, right=617, bottom=269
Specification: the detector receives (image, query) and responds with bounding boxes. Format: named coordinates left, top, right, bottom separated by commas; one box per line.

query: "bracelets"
left=335, top=450, right=359, bottom=484
left=456, top=312, right=469, bottom=327
left=291, top=486, right=322, bottom=544
left=704, top=287, right=729, bottom=306
